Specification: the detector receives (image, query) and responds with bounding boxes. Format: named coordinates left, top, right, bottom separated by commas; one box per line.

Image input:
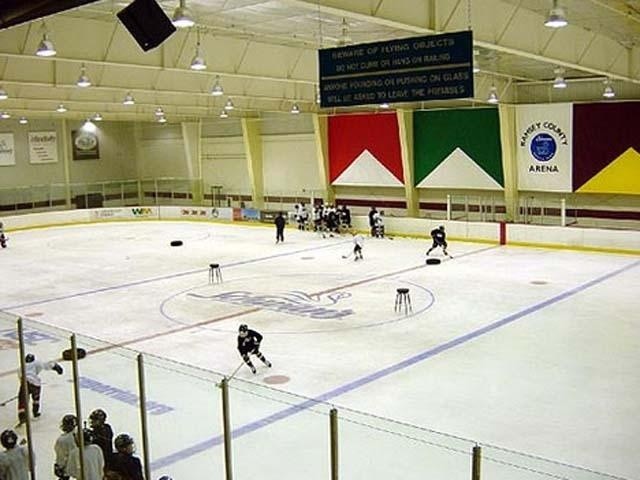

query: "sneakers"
left=265, top=360, right=271, bottom=367
left=251, top=366, right=256, bottom=373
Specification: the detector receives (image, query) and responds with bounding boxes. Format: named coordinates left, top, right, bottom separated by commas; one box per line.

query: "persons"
left=294, top=201, right=385, bottom=238
left=275, top=212, right=285, bottom=243
left=352, top=231, right=364, bottom=261
left=0, top=409, right=174, bottom=480
left=17, top=354, right=62, bottom=423
left=237, top=324, right=271, bottom=373
left=426, top=226, right=448, bottom=255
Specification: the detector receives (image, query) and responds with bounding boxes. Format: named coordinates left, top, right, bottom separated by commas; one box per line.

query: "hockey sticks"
left=224, top=349, right=255, bottom=382
left=0, top=395, right=21, bottom=407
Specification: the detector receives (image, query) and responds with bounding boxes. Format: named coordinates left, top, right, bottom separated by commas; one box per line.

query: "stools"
left=394, top=288, right=413, bottom=316
left=209, top=264, right=222, bottom=284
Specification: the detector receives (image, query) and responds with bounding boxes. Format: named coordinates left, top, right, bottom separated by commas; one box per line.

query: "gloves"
left=52, top=363, right=63, bottom=374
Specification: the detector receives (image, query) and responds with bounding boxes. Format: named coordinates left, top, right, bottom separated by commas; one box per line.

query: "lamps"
left=17, top=116, right=30, bottom=124
left=189, top=47, right=208, bottom=71
left=551, top=69, right=569, bottom=90
left=1, top=88, right=8, bottom=102
left=211, top=76, right=225, bottom=97
left=472, top=60, right=481, bottom=77
left=219, top=110, right=229, bottom=119
left=84, top=111, right=104, bottom=124
left=154, top=107, right=168, bottom=124
left=33, top=32, right=59, bottom=59
left=601, top=83, right=615, bottom=100
left=379, top=103, right=390, bottom=110
left=55, top=103, right=68, bottom=115
left=121, top=92, right=136, bottom=105
left=170, top=1, right=197, bottom=30
left=223, top=99, right=235, bottom=111
left=288, top=102, right=302, bottom=116
left=1, top=109, right=10, bottom=120
left=542, top=2, right=571, bottom=32
left=75, top=62, right=93, bottom=88
left=484, top=85, right=501, bottom=104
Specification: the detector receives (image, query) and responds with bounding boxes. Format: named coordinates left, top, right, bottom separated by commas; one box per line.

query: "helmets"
left=59, top=409, right=137, bottom=456
left=25, top=354, right=35, bottom=362
left=1, top=430, right=17, bottom=449
left=239, top=325, right=249, bottom=337
